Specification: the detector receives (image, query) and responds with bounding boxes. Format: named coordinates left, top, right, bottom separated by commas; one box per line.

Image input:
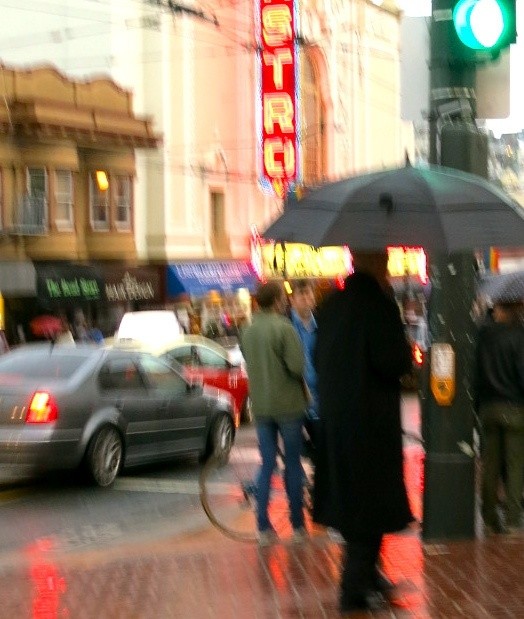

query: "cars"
left=100, top=310, right=253, bottom=430
left=399, top=316, right=428, bottom=388
left=0, top=343, right=236, bottom=488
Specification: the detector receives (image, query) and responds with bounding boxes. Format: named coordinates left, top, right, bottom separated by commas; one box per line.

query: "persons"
left=311, top=235, right=417, bottom=612
left=471, top=299, right=524, bottom=534
left=288, top=279, right=322, bottom=468
left=239, top=280, right=312, bottom=546
left=466, top=291, right=493, bottom=419
left=403, top=298, right=431, bottom=388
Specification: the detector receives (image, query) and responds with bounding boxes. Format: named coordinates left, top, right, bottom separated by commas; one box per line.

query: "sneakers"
left=295, top=527, right=310, bottom=545
left=259, top=530, right=281, bottom=548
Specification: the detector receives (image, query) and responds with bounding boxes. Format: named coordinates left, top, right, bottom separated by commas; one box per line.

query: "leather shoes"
left=363, top=586, right=386, bottom=613
left=374, top=570, right=395, bottom=590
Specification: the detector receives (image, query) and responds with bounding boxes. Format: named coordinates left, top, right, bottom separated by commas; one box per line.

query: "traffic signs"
left=399, top=15, right=510, bottom=121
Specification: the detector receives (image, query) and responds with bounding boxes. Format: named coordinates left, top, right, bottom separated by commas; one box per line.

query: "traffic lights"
left=448, top=0, right=517, bottom=63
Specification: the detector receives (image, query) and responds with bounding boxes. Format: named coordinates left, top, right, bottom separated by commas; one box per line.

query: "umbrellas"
left=486, top=270, right=524, bottom=304
left=259, top=144, right=523, bottom=323
